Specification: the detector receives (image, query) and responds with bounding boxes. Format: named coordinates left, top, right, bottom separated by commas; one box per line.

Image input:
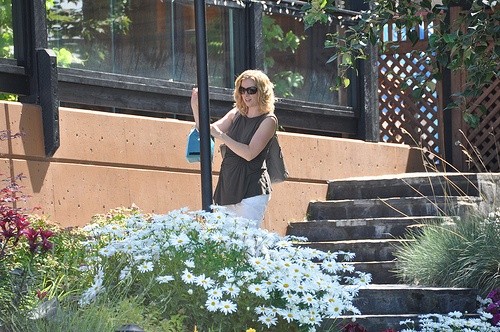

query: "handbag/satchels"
left=266, top=133, right=290, bottom=184
left=186, top=128, right=216, bottom=163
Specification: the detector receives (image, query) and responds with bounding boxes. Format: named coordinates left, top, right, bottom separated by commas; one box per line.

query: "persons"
left=190, top=68, right=280, bottom=230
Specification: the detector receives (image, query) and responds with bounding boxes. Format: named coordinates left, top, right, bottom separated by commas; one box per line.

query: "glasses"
left=237, top=85, right=259, bottom=95
